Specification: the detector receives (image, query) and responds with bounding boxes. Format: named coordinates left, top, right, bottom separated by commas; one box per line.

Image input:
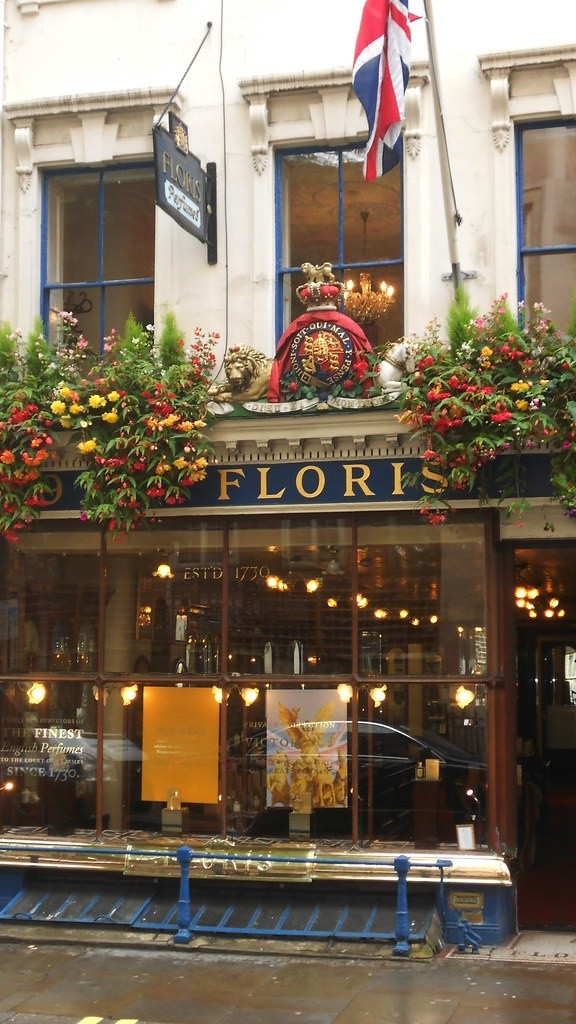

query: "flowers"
left=0, top=308, right=230, bottom=544
left=376, top=299, right=576, bottom=528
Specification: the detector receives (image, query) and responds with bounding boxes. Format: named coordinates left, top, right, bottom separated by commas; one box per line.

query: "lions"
left=208, top=346, right=274, bottom=404
left=301, top=262, right=336, bottom=283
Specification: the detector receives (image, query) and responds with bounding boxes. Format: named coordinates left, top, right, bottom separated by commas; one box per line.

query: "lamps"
left=212, top=684, right=260, bottom=706
left=321, top=549, right=346, bottom=576
left=152, top=548, right=175, bottom=580
left=343, top=211, right=396, bottom=322
left=455, top=686, right=484, bottom=710
left=93, top=684, right=138, bottom=706
left=18, top=682, right=46, bottom=705
left=337, top=684, right=388, bottom=708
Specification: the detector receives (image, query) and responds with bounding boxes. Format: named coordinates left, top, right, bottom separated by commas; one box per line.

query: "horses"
left=374, top=341, right=419, bottom=394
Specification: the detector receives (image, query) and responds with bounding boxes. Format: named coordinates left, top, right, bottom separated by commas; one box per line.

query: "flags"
left=351, top=0, right=423, bottom=182
